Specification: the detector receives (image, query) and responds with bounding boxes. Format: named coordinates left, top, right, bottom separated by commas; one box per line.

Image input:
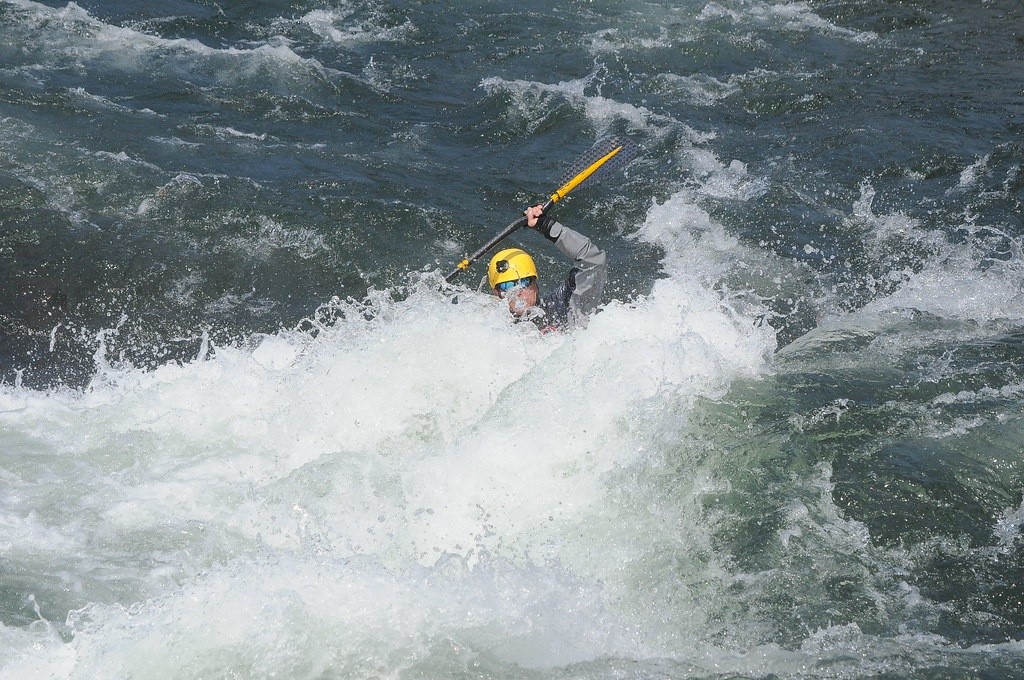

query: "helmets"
left=487, top=248, right=538, bottom=289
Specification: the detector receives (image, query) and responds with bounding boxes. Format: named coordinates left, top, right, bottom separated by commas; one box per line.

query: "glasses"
left=495, top=276, right=536, bottom=292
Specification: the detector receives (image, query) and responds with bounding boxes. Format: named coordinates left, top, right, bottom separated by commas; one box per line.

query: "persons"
left=472, top=205, right=607, bottom=338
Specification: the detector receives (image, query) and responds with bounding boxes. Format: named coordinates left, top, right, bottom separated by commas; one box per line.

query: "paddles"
left=442, top=132, right=640, bottom=281
